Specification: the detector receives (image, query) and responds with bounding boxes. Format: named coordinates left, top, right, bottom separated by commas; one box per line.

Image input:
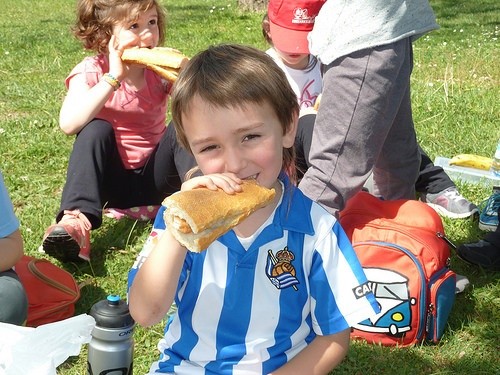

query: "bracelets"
left=101, top=72, right=121, bottom=90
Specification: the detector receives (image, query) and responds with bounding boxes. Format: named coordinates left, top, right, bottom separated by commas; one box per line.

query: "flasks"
left=87, top=294, right=134, bottom=375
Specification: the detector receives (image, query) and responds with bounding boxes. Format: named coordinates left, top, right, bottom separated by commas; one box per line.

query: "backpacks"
left=336, top=191, right=457, bottom=348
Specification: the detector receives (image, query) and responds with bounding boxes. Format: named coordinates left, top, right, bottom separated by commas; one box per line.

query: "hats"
left=268, top=0, right=327, bottom=54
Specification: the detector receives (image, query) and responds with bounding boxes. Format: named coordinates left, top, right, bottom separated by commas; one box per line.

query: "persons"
left=478, top=140, right=500, bottom=231
left=455, top=207, right=500, bottom=269
left=42, top=0, right=204, bottom=265
left=295, top=0, right=440, bottom=216
left=128, top=46, right=381, bottom=375
left=0, top=170, right=29, bottom=327
left=262, top=0, right=477, bottom=218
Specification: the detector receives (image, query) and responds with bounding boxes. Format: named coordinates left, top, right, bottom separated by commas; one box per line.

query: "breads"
left=162, top=177, right=275, bottom=254
left=119, top=48, right=190, bottom=83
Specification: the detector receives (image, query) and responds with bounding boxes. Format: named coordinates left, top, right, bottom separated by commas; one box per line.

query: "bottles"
left=486, top=142, right=500, bottom=189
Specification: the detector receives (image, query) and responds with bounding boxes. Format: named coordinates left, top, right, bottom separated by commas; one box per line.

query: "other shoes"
left=478, top=188, right=500, bottom=231
left=419, top=186, right=478, bottom=218
left=455, top=273, right=469, bottom=294
left=42, top=208, right=92, bottom=265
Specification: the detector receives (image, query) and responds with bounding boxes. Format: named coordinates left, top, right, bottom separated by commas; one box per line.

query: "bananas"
left=448, top=154, right=500, bottom=170
